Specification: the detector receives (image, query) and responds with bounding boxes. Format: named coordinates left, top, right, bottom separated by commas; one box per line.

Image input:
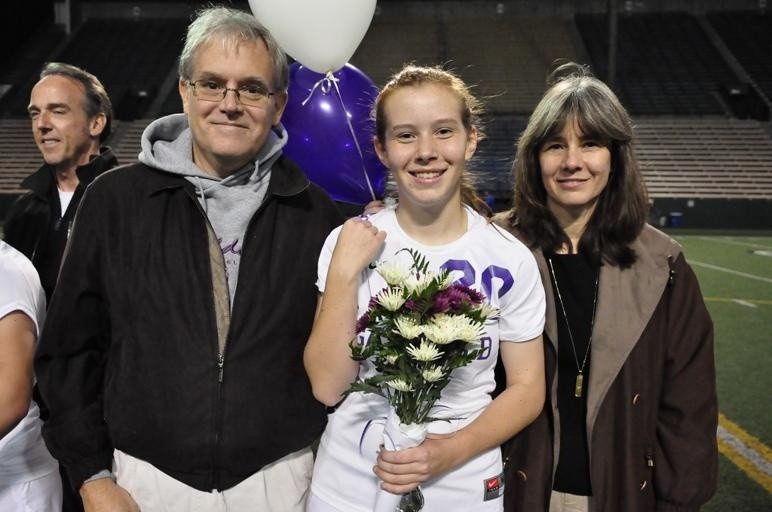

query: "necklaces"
left=549, top=250, right=598, bottom=398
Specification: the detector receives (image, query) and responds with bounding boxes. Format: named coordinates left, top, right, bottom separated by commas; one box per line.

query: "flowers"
left=341, top=242, right=505, bottom=511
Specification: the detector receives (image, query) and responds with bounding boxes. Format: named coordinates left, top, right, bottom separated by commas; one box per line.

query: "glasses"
left=189, top=79, right=275, bottom=107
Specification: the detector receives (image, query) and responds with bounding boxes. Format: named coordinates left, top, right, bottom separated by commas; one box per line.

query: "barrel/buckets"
left=671, top=212, right=683, bottom=228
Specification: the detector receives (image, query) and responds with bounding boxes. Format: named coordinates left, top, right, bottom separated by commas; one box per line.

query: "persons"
left=274, top=59, right=387, bottom=204
left=245, top=0, right=378, bottom=75
left=0, top=238, right=65, bottom=512
left=0, top=61, right=123, bottom=314
left=304, top=63, right=550, bottom=512
left=34, top=1, right=348, bottom=512
left=362, top=60, right=721, bottom=512
